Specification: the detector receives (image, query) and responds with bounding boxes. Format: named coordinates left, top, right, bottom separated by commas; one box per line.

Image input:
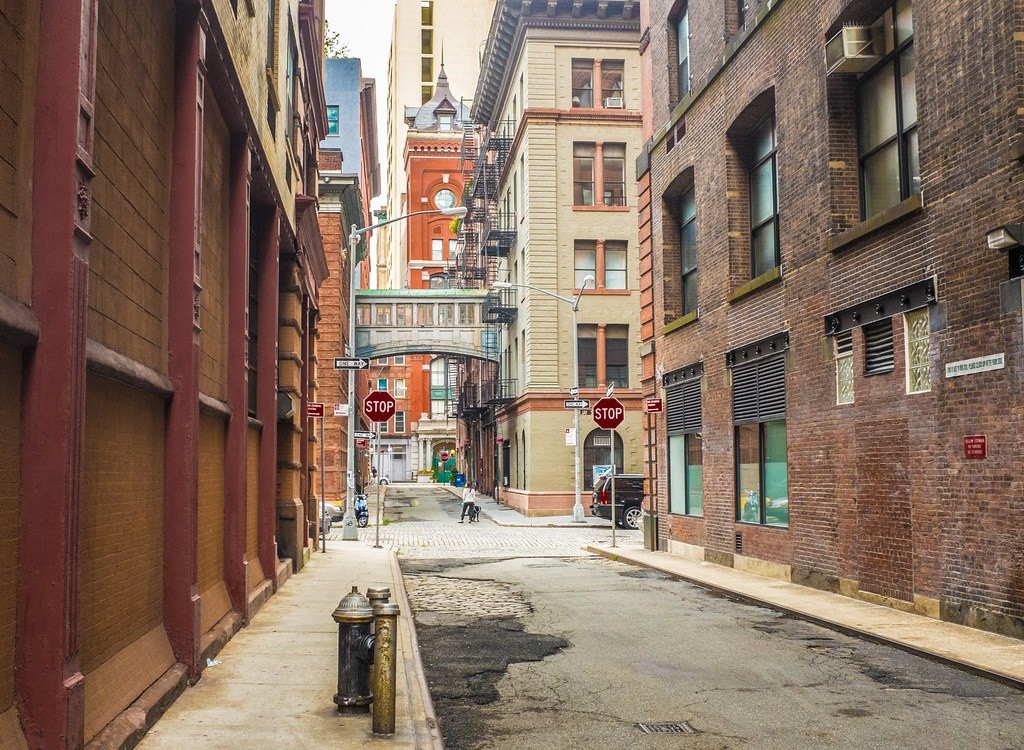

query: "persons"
left=457, top=481, right=475, bottom=523
left=371, top=466, right=377, bottom=486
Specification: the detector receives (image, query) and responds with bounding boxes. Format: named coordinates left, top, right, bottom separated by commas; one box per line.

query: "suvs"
left=588, top=474, right=645, bottom=530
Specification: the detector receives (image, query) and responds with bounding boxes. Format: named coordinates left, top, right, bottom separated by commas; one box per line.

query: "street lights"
left=492, top=275, right=595, bottom=524
left=341, top=206, right=468, bottom=542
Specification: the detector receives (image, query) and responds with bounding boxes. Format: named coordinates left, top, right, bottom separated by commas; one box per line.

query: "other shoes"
left=469, top=521, right=471, bottom=523
left=458, top=522, right=463, bottom=523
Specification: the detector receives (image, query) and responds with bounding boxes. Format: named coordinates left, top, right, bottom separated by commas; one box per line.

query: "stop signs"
left=592, top=397, right=625, bottom=431
left=363, top=390, right=396, bottom=424
left=440, top=453, right=449, bottom=462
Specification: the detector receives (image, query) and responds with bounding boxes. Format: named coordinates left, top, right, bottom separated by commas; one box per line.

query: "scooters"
left=354, top=490, right=372, bottom=527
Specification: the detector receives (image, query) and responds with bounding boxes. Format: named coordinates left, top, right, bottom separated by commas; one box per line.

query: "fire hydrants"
left=331, top=585, right=376, bottom=715
